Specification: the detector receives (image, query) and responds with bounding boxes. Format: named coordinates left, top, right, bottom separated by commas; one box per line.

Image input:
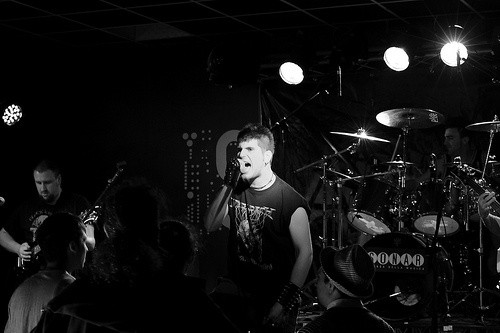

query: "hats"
left=319, top=243, right=376, bottom=299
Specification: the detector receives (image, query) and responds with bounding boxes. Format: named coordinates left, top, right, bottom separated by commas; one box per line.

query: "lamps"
left=382, top=42, right=415, bottom=72
left=278, top=48, right=313, bottom=87
left=440, top=30, right=470, bottom=66
left=0, top=101, right=24, bottom=126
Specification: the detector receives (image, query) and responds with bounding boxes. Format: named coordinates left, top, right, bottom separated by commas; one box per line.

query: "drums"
left=357, top=233, right=454, bottom=321
left=348, top=191, right=403, bottom=236
left=412, top=191, right=463, bottom=236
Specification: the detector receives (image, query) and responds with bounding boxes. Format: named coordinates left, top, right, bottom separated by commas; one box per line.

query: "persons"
left=203, top=125, right=313, bottom=333
left=430, top=118, right=500, bottom=245
left=299, top=246, right=397, bottom=333
left=0, top=161, right=96, bottom=332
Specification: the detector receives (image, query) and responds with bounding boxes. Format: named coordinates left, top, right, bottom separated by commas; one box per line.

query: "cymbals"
left=465, top=120, right=500, bottom=133
left=329, top=129, right=391, bottom=144
left=319, top=132, right=356, bottom=171
left=376, top=107, right=444, bottom=129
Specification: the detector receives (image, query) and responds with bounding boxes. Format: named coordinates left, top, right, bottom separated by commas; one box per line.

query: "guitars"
left=452, top=166, right=500, bottom=215
left=12, top=208, right=99, bottom=287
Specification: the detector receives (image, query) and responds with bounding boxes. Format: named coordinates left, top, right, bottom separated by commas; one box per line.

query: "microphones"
left=228, top=158, right=246, bottom=172
left=337, top=65, right=343, bottom=96
